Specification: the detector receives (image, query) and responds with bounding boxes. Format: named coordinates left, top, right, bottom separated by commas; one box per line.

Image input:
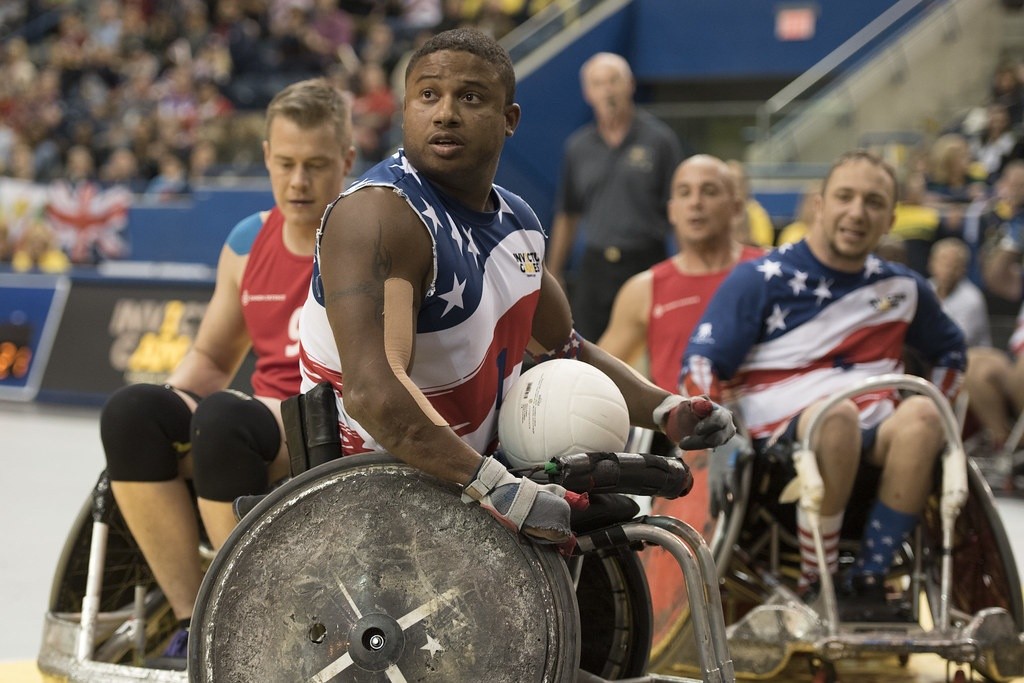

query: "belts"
left=583, top=245, right=642, bottom=263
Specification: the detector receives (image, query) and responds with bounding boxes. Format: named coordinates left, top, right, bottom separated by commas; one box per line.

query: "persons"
left=593, top=155, right=769, bottom=390
left=726, top=45, right=1024, bottom=458
left=544, top=51, right=685, bottom=347
left=0, top=1, right=559, bottom=198
left=101, top=83, right=356, bottom=659
left=678, top=149, right=969, bottom=620
left=301, top=29, right=736, bottom=548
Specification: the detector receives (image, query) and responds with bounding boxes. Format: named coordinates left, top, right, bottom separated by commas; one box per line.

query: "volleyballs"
left=496, top=357, right=632, bottom=472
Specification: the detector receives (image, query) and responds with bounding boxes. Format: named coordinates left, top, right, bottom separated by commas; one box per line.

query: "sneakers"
left=143, top=630, right=189, bottom=671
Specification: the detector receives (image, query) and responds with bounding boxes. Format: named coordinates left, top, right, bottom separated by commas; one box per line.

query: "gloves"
left=652, top=394, right=737, bottom=451
left=460, top=455, right=590, bottom=545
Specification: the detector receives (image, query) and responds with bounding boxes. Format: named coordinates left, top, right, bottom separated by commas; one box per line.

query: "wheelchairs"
left=35, top=469, right=193, bottom=683
left=637, top=375, right=1024, bottom=683
left=187, top=379, right=737, bottom=683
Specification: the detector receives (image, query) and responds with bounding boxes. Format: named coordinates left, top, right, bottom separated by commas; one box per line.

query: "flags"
left=37, top=175, right=135, bottom=268
